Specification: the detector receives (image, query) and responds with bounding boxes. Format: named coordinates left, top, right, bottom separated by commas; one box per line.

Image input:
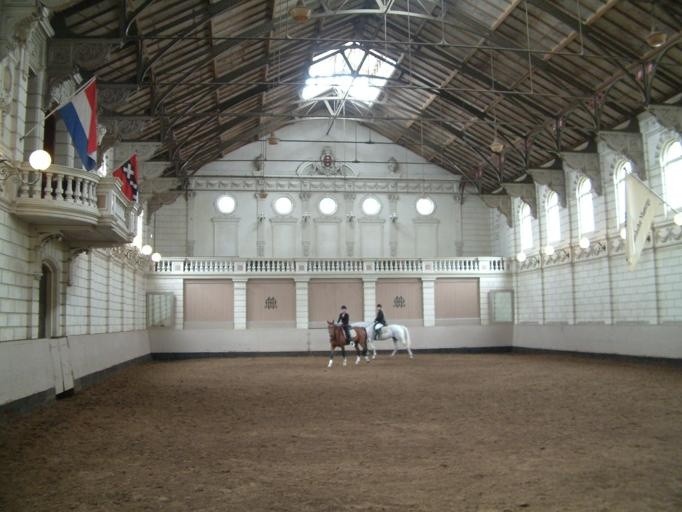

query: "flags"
left=112, top=152, right=140, bottom=203
left=55, top=77, right=98, bottom=173
left=625, top=171, right=661, bottom=270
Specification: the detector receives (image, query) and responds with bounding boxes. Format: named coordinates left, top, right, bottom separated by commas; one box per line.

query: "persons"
left=371, top=303, right=387, bottom=338
left=335, top=305, right=352, bottom=344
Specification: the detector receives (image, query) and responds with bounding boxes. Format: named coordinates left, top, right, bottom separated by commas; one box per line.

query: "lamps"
left=289, top=0, right=312, bottom=22
left=404, top=0, right=418, bottom=90
left=438, top=0, right=451, bottom=49
left=259, top=116, right=268, bottom=198
left=645, top=0, right=666, bottom=47
left=0, top=150, right=52, bottom=184
left=489, top=42, right=504, bottom=153
left=139, top=245, right=161, bottom=266
left=268, top=37, right=280, bottom=144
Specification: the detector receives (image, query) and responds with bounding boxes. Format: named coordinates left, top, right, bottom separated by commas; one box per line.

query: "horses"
left=327, top=320, right=368, bottom=368
left=365, top=324, right=414, bottom=360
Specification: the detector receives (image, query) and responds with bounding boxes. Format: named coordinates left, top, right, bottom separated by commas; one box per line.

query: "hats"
left=377, top=304, right=382, bottom=308
left=341, top=305, right=346, bottom=309
